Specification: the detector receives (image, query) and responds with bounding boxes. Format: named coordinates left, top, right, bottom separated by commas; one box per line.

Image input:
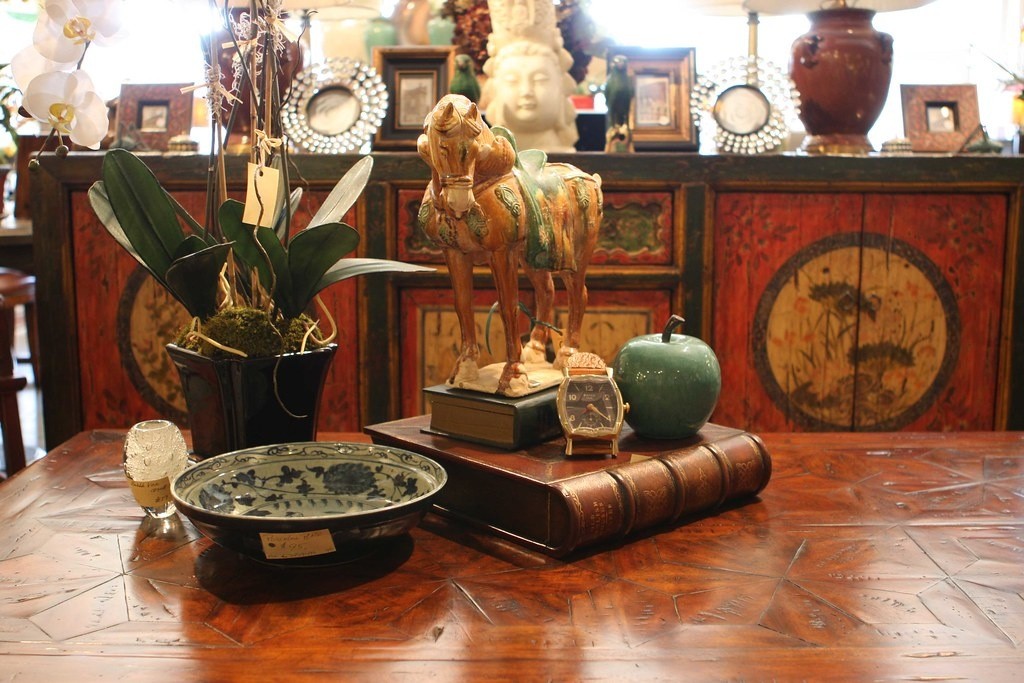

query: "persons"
left=483, top=2, right=578, bottom=153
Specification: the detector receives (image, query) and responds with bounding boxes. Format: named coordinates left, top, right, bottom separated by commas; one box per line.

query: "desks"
left=1, top=428, right=1021, bottom=681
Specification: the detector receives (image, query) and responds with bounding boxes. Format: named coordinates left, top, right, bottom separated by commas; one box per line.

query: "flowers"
left=10, top=0, right=129, bottom=169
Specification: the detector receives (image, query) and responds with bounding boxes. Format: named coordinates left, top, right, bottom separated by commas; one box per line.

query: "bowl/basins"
left=169, top=439, right=448, bottom=565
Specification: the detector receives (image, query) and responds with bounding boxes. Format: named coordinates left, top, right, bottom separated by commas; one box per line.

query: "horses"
left=416, top=94, right=604, bottom=401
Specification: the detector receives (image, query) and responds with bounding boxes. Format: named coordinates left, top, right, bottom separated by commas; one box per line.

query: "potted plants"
left=84, top=147, right=442, bottom=460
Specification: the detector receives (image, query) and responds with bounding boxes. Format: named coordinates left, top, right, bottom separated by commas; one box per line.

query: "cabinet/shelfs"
left=28, top=148, right=1023, bottom=435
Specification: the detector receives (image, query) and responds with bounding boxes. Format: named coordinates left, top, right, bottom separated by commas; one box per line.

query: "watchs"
left=556, top=351, right=631, bottom=457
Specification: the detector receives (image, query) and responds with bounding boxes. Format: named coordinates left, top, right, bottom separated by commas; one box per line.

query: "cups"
left=121, top=420, right=188, bottom=520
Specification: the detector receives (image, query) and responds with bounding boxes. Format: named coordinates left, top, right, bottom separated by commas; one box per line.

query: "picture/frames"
left=371, top=43, right=459, bottom=151
left=605, top=45, right=701, bottom=152
left=113, top=83, right=196, bottom=151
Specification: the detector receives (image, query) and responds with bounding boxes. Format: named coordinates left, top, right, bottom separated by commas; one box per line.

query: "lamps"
left=745, top=0, right=933, bottom=156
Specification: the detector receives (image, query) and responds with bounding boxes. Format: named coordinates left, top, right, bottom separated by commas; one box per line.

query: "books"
left=362, top=397, right=773, bottom=557
